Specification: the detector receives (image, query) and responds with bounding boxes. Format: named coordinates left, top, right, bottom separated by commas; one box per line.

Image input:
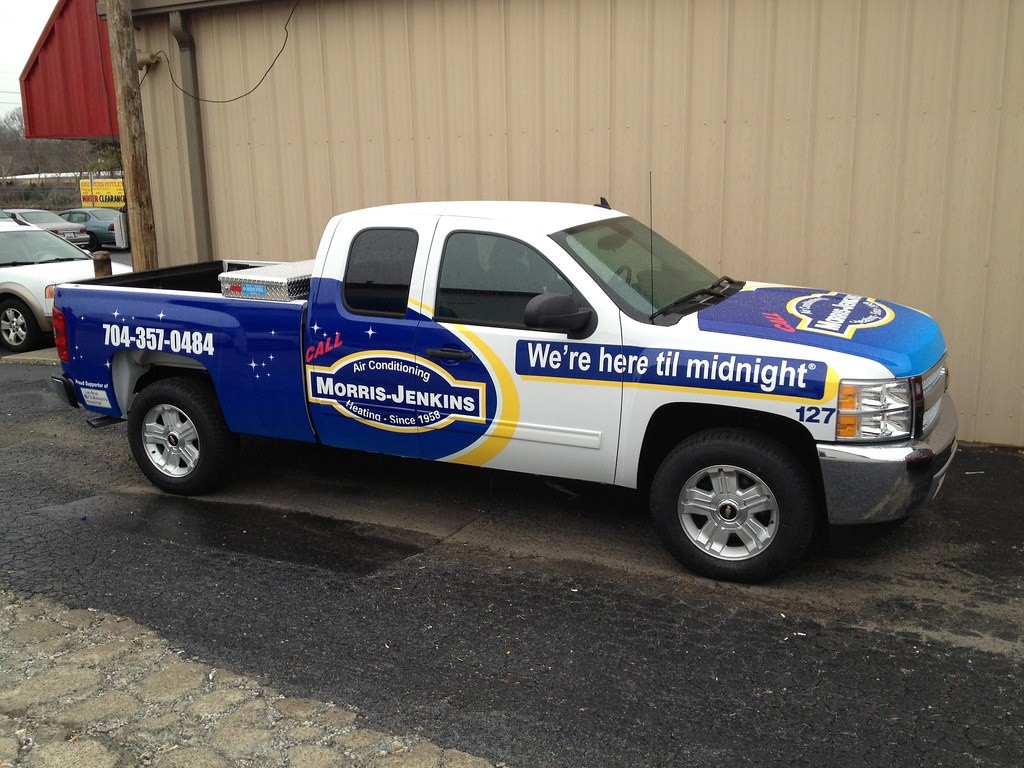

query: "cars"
left=59, top=206, right=123, bottom=251
left=0, top=209, right=132, bottom=354
left=2, top=208, right=90, bottom=248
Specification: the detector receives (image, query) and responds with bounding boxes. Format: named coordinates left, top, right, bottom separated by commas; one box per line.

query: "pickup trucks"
left=50, top=200, right=960, bottom=586
